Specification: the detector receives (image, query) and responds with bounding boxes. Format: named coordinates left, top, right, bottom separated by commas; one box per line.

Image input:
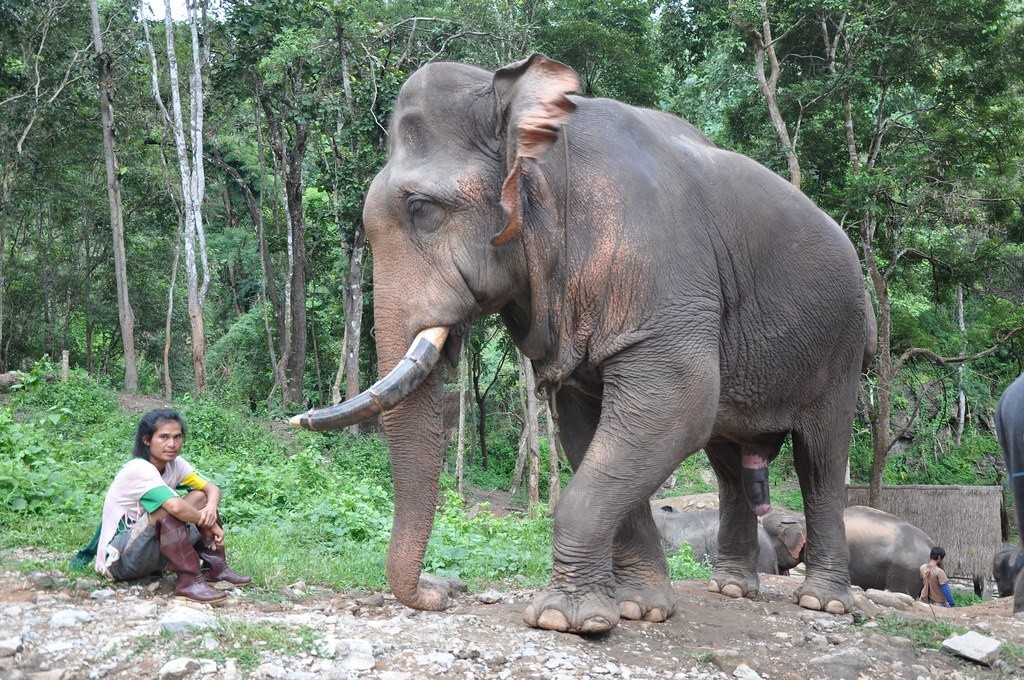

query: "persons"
left=920, top=546, right=956, bottom=608
left=95, top=408, right=251, bottom=603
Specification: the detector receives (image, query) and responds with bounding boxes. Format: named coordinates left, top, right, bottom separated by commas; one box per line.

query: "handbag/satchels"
left=919, top=578, right=929, bottom=603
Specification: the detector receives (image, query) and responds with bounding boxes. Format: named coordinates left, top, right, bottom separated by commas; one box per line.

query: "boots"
left=196, top=509, right=251, bottom=587
left=155, top=513, right=228, bottom=604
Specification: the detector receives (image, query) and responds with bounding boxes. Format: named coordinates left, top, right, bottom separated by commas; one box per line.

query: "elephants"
left=650, top=364, right=1024, bottom=615
left=284, top=51, right=879, bottom=637
